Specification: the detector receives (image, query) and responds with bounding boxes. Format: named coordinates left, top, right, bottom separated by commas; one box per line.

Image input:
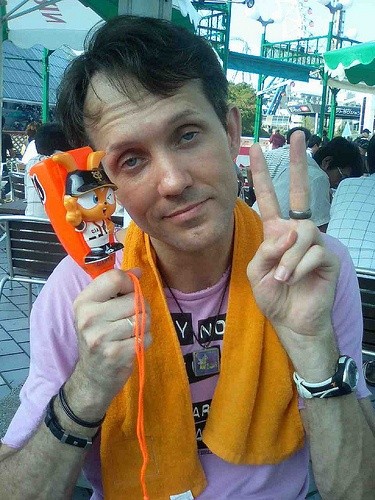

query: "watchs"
left=291, top=354, right=359, bottom=401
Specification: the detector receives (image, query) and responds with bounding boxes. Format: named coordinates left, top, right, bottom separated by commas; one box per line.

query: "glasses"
left=336, top=163, right=350, bottom=180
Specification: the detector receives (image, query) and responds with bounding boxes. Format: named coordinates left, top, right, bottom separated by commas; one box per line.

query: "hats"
left=362, top=129, right=371, bottom=133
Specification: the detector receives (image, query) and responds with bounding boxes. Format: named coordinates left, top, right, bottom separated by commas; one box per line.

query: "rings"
left=289, top=209, right=312, bottom=223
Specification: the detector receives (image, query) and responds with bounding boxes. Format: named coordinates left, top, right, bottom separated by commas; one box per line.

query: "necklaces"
left=154, top=260, right=231, bottom=377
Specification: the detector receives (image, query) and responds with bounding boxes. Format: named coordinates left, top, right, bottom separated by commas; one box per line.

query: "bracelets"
left=42, top=396, right=97, bottom=448
left=59, top=383, right=106, bottom=427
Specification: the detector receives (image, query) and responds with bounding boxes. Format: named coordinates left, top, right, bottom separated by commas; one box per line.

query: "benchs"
left=0, top=216, right=68, bottom=326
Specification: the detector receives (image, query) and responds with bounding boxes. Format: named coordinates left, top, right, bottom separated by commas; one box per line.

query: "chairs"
left=9, top=171, right=27, bottom=203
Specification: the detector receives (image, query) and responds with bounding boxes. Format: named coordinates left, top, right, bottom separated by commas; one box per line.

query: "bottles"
left=1, top=164, right=13, bottom=203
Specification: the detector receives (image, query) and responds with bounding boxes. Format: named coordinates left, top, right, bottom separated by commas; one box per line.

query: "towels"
left=100, top=196, right=305, bottom=500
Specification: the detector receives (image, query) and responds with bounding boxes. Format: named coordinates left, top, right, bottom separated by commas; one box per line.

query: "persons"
left=253, top=138, right=364, bottom=231
left=0, top=116, right=13, bottom=204
left=2, top=16, right=375, bottom=500
left=321, top=136, right=373, bottom=269
left=268, top=125, right=375, bottom=170
left=23, top=123, right=80, bottom=222
